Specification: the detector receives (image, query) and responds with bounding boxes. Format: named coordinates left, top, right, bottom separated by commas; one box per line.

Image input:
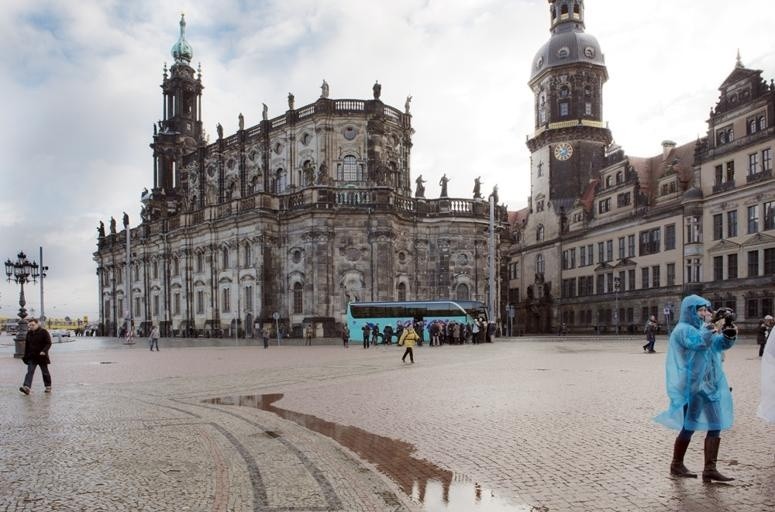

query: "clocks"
left=554, top=143, right=573, bottom=161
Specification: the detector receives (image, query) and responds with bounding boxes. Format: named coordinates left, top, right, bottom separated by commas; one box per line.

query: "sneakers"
left=44, top=385, right=51, bottom=392
left=643, top=345, right=656, bottom=353
left=20, top=387, right=30, bottom=394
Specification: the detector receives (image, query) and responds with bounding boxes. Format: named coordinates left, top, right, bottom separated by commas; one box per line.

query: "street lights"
left=613, top=276, right=621, bottom=335
left=3, top=253, right=44, bottom=357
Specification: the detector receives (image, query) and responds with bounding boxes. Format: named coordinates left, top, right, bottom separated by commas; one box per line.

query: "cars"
left=52, top=329, right=71, bottom=338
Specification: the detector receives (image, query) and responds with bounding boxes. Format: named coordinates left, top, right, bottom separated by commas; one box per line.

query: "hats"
left=764, top=315, right=774, bottom=319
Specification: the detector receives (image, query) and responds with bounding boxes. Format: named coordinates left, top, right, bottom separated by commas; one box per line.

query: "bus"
left=5, top=318, right=29, bottom=335
left=348, top=299, right=490, bottom=343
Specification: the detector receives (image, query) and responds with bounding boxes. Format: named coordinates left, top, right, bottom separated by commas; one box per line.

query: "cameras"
left=715, top=308, right=737, bottom=337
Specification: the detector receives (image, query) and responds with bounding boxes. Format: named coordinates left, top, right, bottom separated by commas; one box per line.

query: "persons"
left=19, top=318, right=52, bottom=395
left=361, top=316, right=497, bottom=349
left=398, top=322, right=423, bottom=364
left=148, top=325, right=160, bottom=352
left=756, top=314, right=774, bottom=356
left=305, top=324, right=314, bottom=346
left=262, top=326, right=271, bottom=350
left=643, top=315, right=659, bottom=353
left=654, top=294, right=735, bottom=484
left=341, top=323, right=350, bottom=346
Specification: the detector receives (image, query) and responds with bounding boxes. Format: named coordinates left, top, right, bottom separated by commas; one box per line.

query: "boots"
left=670, top=437, right=697, bottom=477
left=702, top=438, right=735, bottom=482
